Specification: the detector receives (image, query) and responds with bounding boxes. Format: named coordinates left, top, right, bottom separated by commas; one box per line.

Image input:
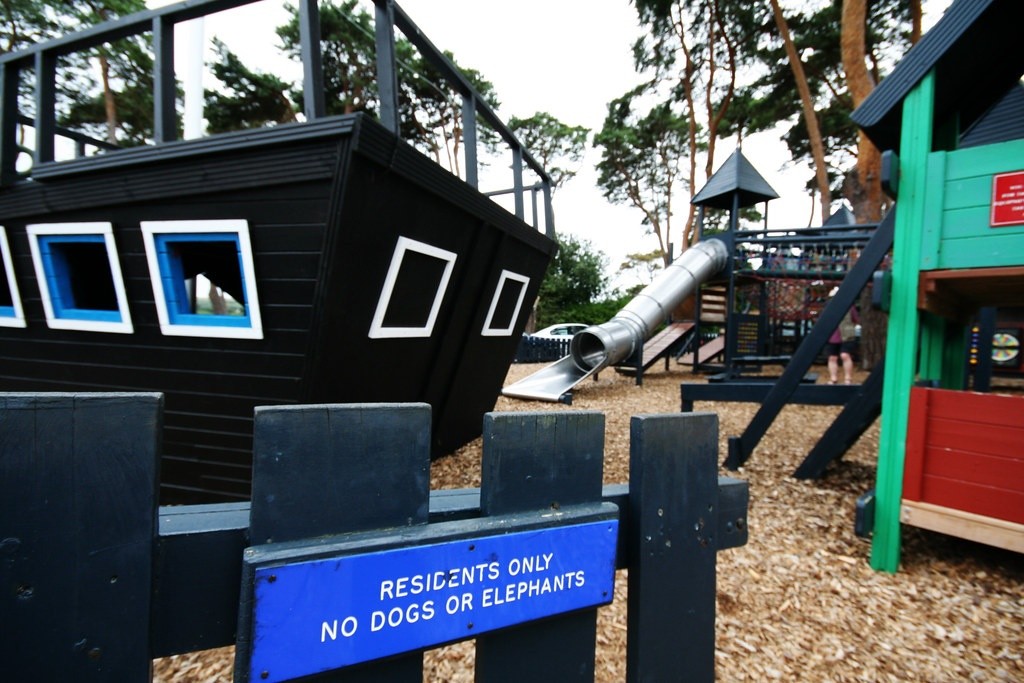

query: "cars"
left=530, top=322, right=591, bottom=359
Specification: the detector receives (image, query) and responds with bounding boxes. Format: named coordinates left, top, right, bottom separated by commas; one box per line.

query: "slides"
left=501, top=238, right=729, bottom=403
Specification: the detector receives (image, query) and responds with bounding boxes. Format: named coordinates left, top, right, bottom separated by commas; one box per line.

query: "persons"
left=826, top=286, right=861, bottom=385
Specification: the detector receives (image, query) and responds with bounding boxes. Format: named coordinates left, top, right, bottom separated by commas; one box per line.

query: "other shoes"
left=826, top=378, right=840, bottom=385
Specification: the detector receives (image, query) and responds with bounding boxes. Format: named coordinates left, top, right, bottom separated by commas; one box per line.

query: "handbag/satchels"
left=839, top=312, right=857, bottom=341
